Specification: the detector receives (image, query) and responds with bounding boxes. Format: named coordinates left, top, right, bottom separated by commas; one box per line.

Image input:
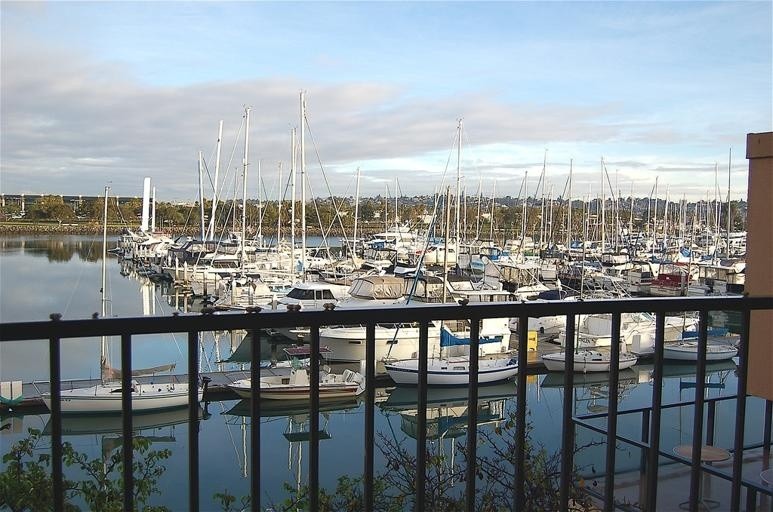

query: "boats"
left=31, top=186, right=205, bottom=414
left=219, top=399, right=360, bottom=420
left=36, top=402, right=206, bottom=504
